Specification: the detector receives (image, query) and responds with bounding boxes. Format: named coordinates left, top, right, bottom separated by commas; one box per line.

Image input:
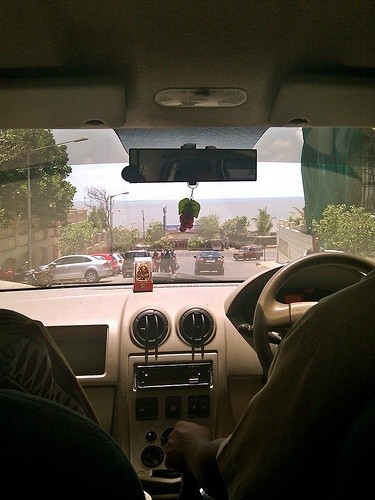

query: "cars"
left=30, top=253, right=124, bottom=287
left=232, top=246, right=263, bottom=260
left=123, top=251, right=155, bottom=277
left=194, top=251, right=225, bottom=276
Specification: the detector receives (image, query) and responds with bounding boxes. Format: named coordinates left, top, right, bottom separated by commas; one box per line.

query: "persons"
left=153, top=251, right=179, bottom=273
left=166, top=272, right=375, bottom=500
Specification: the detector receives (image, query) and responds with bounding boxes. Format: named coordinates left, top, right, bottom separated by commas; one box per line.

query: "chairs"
left=0, top=388, right=146, bottom=500
left=238, top=378, right=375, bottom=500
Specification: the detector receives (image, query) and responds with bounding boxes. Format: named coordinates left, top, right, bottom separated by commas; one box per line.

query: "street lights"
left=26, top=136, right=89, bottom=271
left=110, top=191, right=130, bottom=253
